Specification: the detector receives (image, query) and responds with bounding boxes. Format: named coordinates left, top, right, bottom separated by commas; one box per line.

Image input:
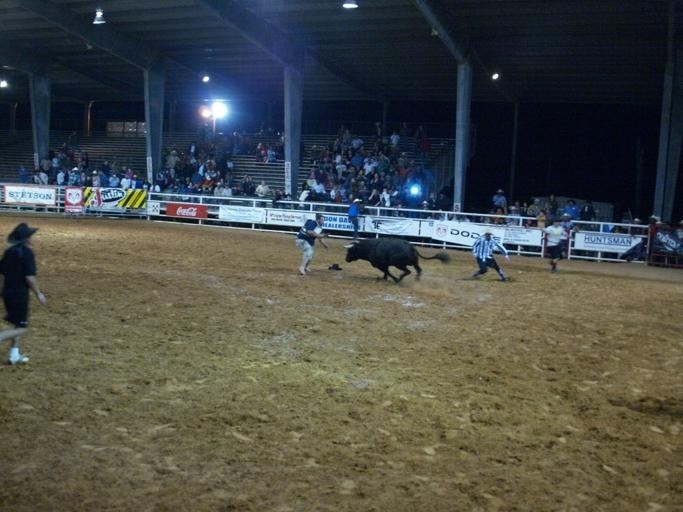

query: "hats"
left=6, top=222, right=38, bottom=244
left=353, top=198, right=363, bottom=203
left=496, top=189, right=504, bottom=193
left=72, top=166, right=79, bottom=171
left=551, top=215, right=562, bottom=222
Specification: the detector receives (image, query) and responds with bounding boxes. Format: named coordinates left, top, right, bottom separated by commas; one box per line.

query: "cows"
left=342, top=238, right=453, bottom=284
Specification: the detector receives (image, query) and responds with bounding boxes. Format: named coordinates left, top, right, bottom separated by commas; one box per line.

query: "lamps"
left=92, top=5, right=106, bottom=25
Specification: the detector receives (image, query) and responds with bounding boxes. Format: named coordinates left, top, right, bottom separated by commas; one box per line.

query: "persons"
left=18, top=145, right=144, bottom=190
left=297, top=119, right=443, bottom=219
left=488, top=188, right=596, bottom=245
left=543, top=218, right=569, bottom=273
left=0, top=221, right=47, bottom=365
left=150, top=120, right=286, bottom=209
left=294, top=214, right=330, bottom=275
left=347, top=198, right=364, bottom=239
left=472, top=231, right=512, bottom=281
left=610, top=214, right=661, bottom=261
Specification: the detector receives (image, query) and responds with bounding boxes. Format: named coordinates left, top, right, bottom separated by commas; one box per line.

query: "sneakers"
left=498, top=271, right=506, bottom=281
left=299, top=266, right=311, bottom=274
left=8, top=353, right=29, bottom=364
left=471, top=271, right=480, bottom=278
left=549, top=260, right=557, bottom=272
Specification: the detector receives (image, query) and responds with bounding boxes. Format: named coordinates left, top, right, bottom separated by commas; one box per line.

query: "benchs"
left=0, top=127, right=456, bottom=215
